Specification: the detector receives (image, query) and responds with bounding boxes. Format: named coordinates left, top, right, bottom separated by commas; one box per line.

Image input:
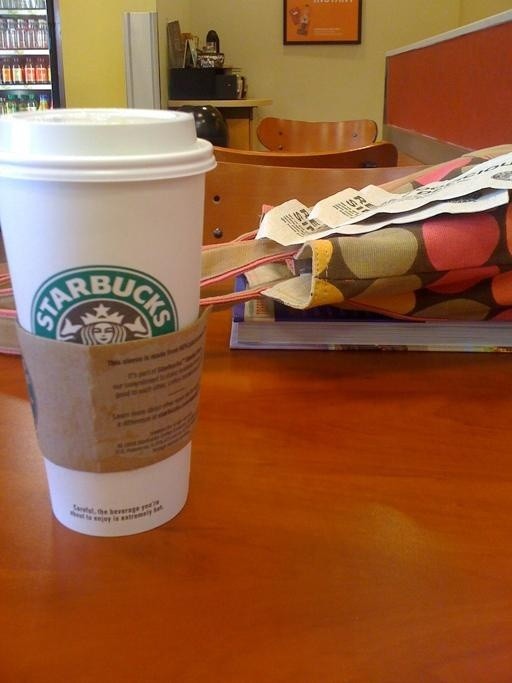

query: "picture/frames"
left=281, top=0, right=363, bottom=47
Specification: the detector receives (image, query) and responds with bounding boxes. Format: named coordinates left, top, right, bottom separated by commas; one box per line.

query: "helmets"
left=177, top=104, right=228, bottom=148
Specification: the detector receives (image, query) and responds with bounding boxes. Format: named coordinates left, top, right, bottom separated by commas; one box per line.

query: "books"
left=226, top=267, right=512, bottom=357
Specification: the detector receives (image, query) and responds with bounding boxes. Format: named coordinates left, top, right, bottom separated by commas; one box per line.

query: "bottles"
left=0, top=107, right=50, bottom=114
left=0, top=93, right=50, bottom=108
left=1, top=18, right=47, bottom=49
left=1, top=56, right=50, bottom=84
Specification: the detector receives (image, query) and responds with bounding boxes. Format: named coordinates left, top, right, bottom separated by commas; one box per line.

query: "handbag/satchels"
left=241, top=142, right=512, bottom=324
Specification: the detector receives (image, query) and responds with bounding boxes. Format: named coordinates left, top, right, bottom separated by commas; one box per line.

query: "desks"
left=2, top=296, right=511, bottom=682
left=167, top=99, right=275, bottom=152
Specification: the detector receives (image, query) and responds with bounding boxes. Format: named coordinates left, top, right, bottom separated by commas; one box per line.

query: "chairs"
left=202, top=161, right=435, bottom=243
left=212, top=142, right=401, bottom=169
left=257, top=116, right=378, bottom=152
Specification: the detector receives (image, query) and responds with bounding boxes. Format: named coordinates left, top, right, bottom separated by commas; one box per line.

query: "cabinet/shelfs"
left=2, top=0, right=66, bottom=115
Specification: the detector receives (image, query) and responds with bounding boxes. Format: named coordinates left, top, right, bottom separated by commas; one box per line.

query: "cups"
left=0, top=107, right=218, bottom=538
